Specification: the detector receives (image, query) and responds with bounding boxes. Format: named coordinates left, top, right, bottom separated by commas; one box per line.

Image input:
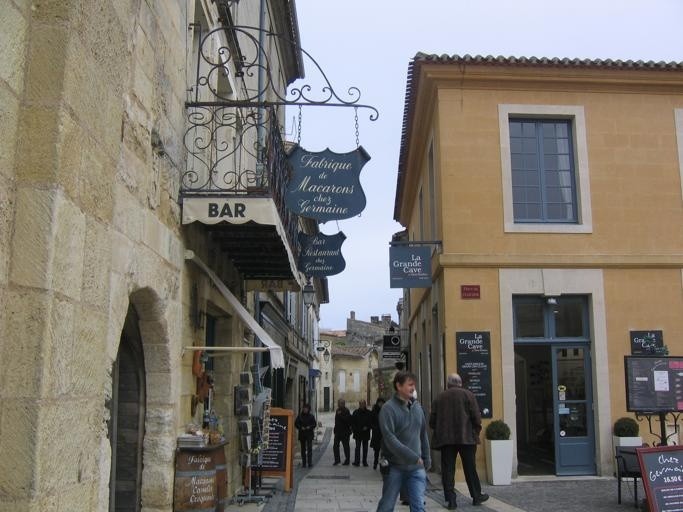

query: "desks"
left=173, top=441, right=230, bottom=511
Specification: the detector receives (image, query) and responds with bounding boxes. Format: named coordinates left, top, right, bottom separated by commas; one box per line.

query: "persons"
left=399, top=387, right=428, bottom=506
left=352, top=399, right=371, bottom=466
left=332, top=398, right=351, bottom=466
left=376, top=370, right=432, bottom=512
left=371, top=396, right=385, bottom=469
left=426, top=373, right=490, bottom=510
left=293, top=402, right=316, bottom=468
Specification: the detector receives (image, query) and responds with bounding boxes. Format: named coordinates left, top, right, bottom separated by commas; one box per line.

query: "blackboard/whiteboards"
left=456, top=331, right=493, bottom=418
left=256, top=407, right=294, bottom=476
left=624, top=330, right=683, bottom=413
left=635, top=445, right=683, bottom=512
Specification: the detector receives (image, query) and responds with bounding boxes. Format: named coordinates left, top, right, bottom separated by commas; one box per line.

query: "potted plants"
left=612, top=417, right=642, bottom=478
left=484, top=419, right=513, bottom=486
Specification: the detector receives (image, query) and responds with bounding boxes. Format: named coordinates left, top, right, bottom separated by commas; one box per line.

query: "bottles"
left=202, top=409, right=217, bottom=434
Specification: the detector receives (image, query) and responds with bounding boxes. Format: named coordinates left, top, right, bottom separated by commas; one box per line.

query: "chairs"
left=616, top=445, right=651, bottom=508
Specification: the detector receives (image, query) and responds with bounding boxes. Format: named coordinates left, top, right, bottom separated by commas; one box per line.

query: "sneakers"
left=351, top=460, right=378, bottom=466
left=446, top=499, right=457, bottom=508
left=302, top=463, right=312, bottom=467
left=332, top=459, right=341, bottom=465
left=400, top=497, right=425, bottom=505
left=342, top=459, right=349, bottom=465
left=471, top=492, right=489, bottom=505
